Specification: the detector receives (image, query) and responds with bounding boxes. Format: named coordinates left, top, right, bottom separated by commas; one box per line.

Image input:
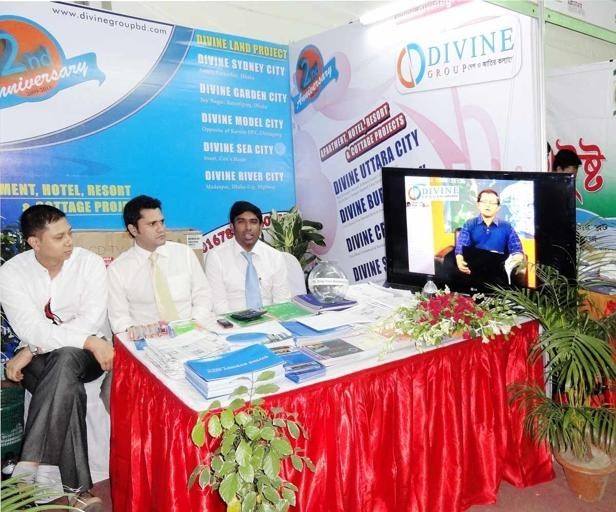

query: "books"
left=139, top=281, right=416, bottom=398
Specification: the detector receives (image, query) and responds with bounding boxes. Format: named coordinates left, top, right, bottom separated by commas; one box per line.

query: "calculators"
left=230, top=308, right=267, bottom=320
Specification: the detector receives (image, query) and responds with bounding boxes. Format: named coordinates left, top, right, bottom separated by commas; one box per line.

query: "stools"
left=24, top=370, right=110, bottom=503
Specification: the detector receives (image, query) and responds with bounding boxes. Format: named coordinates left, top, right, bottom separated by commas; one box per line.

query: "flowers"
left=396, top=285, right=511, bottom=348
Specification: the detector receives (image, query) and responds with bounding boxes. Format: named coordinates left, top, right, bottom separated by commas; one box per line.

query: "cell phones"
left=217, top=318, right=233, bottom=328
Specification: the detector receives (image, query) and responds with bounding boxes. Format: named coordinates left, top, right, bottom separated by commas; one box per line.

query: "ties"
left=149, top=251, right=181, bottom=323
left=242, top=251, right=263, bottom=309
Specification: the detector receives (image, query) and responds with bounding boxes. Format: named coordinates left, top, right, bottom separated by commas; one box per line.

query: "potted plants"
left=486, top=261, right=616, bottom=502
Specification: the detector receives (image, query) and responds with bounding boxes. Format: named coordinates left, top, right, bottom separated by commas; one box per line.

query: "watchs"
left=28, top=343, right=40, bottom=358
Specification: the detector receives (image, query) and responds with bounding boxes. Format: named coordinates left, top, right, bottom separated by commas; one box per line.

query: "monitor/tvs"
left=380, top=165, right=577, bottom=305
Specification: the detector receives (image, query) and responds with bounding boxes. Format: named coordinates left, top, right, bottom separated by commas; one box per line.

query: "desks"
left=111, top=284, right=554, bottom=512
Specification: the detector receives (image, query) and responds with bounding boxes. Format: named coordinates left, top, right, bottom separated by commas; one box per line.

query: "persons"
left=552, top=149, right=582, bottom=180
left=1, top=204, right=116, bottom=510
left=206, top=200, right=290, bottom=308
left=97, top=196, right=212, bottom=417
left=441, top=188, right=525, bottom=288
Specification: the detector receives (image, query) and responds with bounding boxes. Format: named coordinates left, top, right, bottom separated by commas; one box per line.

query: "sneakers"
left=69, top=490, right=104, bottom=512
